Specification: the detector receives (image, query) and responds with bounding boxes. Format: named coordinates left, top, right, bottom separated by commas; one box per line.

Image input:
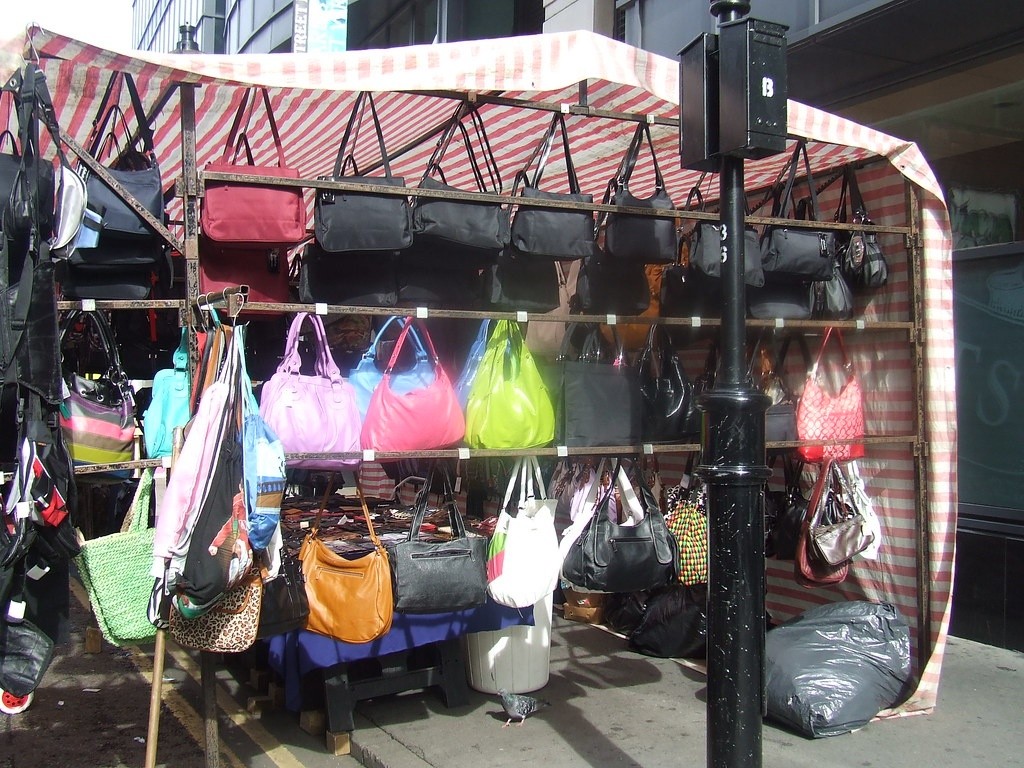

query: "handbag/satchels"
left=0, top=66, right=888, bottom=703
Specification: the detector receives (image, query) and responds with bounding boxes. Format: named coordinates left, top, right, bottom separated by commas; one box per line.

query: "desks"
left=260, top=552, right=535, bottom=732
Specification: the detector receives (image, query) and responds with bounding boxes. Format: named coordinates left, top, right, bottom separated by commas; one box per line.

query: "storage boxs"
left=563, top=591, right=609, bottom=625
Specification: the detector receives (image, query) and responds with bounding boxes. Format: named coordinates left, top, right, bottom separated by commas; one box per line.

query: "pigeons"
left=497, top=688, right=552, bottom=728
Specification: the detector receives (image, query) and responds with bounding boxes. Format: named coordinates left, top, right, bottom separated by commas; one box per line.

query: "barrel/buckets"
left=465, top=591, right=553, bottom=694
